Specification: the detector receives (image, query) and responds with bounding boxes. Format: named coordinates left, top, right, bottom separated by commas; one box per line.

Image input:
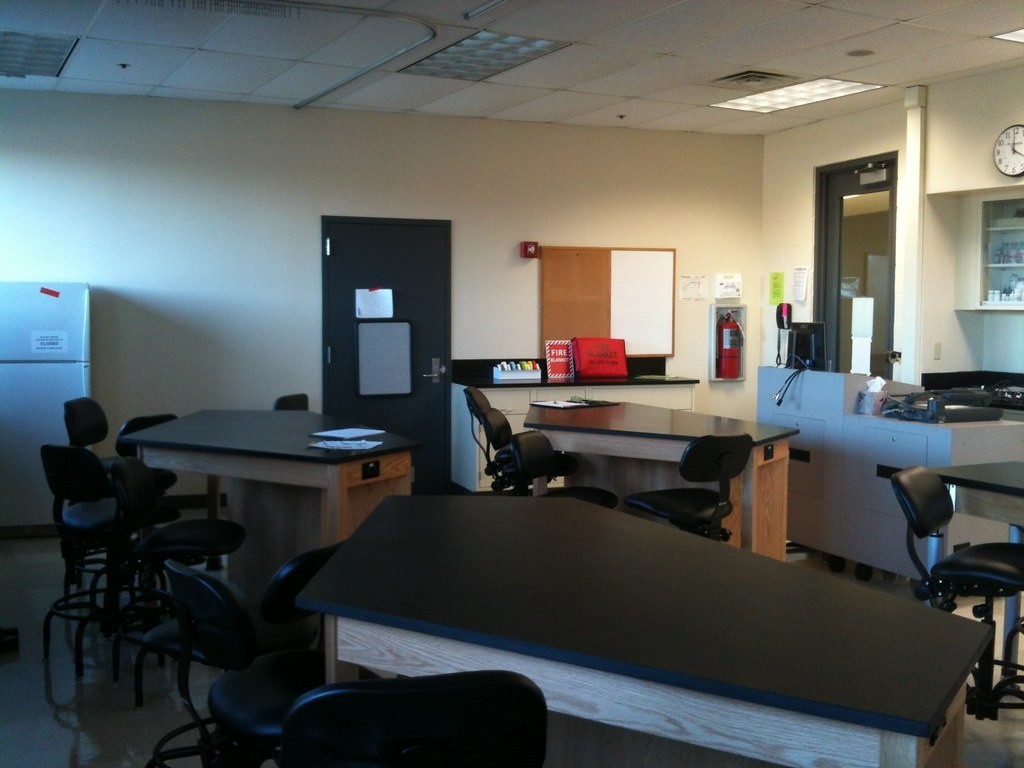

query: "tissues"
left=857, top=375, right=888, bottom=416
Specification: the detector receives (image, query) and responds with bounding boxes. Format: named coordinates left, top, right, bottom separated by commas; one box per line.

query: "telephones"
left=776, top=302, right=792, bottom=330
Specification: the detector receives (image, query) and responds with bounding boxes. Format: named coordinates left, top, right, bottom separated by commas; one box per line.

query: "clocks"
left=992, top=125, right=1024, bottom=178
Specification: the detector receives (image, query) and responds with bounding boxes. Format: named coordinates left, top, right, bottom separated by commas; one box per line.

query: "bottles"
left=990, top=240, right=1024, bottom=264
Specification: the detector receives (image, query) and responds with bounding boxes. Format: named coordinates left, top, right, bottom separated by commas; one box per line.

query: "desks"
left=116, top=411, right=423, bottom=657
left=524, top=401, right=800, bottom=563
left=877, top=462, right=1024, bottom=689
left=293, top=493, right=993, bottom=768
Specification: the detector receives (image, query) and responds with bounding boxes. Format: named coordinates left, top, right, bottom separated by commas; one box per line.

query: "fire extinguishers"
left=716, top=311, right=741, bottom=379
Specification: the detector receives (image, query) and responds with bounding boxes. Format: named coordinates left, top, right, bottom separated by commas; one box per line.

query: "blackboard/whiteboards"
left=611, top=246, right=677, bottom=358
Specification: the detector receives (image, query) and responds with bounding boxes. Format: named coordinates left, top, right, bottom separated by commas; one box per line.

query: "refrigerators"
left=0, top=281, right=92, bottom=538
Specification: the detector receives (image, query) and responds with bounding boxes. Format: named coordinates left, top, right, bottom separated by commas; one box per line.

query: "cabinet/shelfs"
left=449, top=377, right=699, bottom=495
left=953, top=186, right=1024, bottom=311
left=757, top=368, right=1024, bottom=594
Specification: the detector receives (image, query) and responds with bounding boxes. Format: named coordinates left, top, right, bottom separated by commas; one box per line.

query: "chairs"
left=892, top=467, right=1024, bottom=722
left=39, top=385, right=620, bottom=768
left=624, top=432, right=754, bottom=543
